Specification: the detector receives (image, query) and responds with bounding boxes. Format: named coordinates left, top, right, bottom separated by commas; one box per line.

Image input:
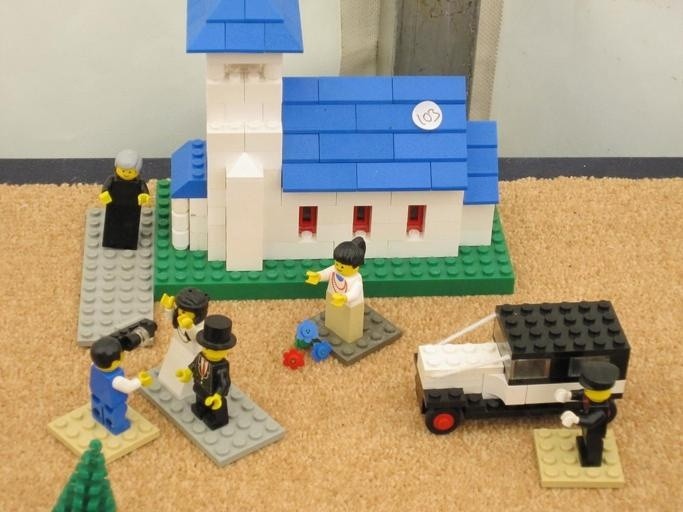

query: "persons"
left=155, top=285, right=209, bottom=400
left=99, top=148, right=154, bottom=250
left=175, top=316, right=239, bottom=431
left=305, top=236, right=366, bottom=343
left=46, top=0, right=631, bottom=512
left=86, top=336, right=152, bottom=434
left=553, top=359, right=619, bottom=467
